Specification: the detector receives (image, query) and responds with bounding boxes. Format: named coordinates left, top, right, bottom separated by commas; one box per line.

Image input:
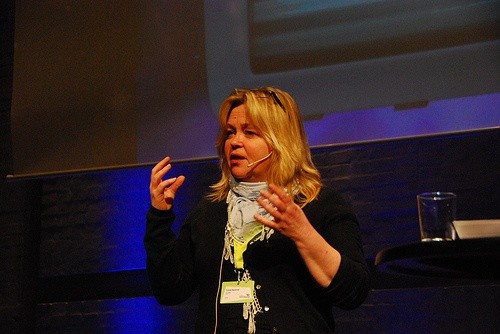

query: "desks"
left=375, top=236, right=500, bottom=284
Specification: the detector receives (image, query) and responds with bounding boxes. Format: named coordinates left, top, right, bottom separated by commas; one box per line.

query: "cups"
left=417, top=192, right=456, bottom=242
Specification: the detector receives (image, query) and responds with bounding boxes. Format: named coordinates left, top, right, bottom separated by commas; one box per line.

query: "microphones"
left=247, top=152, right=272, bottom=167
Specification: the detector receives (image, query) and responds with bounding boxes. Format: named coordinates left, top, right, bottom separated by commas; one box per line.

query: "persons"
left=144, top=86, right=372, bottom=334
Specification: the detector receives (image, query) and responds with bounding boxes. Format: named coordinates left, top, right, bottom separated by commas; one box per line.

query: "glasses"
left=228, top=86, right=286, bottom=114
